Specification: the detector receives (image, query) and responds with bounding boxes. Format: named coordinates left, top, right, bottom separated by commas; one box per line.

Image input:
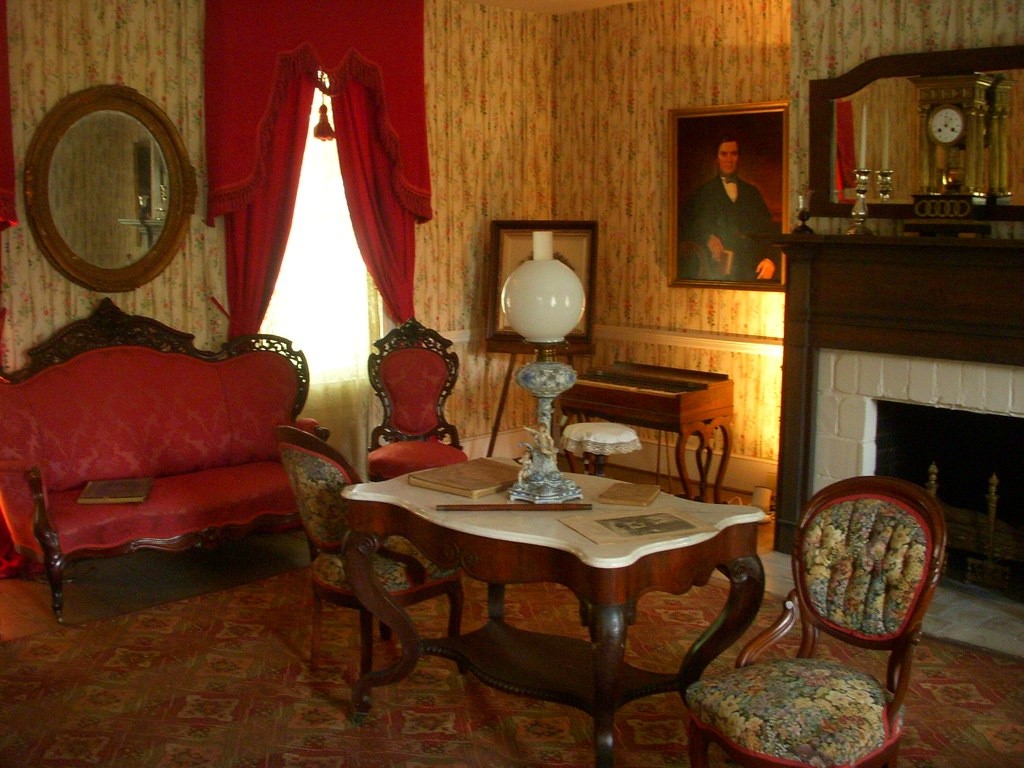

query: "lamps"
left=500, top=231, right=585, bottom=505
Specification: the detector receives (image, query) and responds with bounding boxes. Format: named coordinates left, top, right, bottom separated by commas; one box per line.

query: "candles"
left=882, top=108, right=889, bottom=169
left=860, top=101, right=867, bottom=169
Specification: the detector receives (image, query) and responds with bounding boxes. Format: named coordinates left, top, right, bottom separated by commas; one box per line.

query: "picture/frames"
left=483, top=220, right=598, bottom=354
left=666, top=101, right=791, bottom=290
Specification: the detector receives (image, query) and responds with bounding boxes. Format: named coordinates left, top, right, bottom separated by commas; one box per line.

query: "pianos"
left=560, top=359, right=735, bottom=505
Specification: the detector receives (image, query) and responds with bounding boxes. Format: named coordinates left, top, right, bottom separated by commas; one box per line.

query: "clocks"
left=986, top=71, right=1016, bottom=205
left=902, top=67, right=991, bottom=237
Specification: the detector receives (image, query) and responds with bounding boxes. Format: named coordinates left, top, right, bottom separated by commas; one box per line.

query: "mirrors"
left=23, top=84, right=198, bottom=293
left=808, top=46, right=1024, bottom=222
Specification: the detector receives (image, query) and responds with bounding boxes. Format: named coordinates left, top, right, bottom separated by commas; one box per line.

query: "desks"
left=340, top=459, right=771, bottom=768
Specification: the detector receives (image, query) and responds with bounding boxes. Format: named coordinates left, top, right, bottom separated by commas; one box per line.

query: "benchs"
left=0, top=297, right=330, bottom=624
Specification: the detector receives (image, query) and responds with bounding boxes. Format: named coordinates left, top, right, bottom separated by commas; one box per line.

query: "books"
left=407, top=456, right=524, bottom=498
left=597, top=482, right=662, bottom=506
left=76, top=478, right=150, bottom=503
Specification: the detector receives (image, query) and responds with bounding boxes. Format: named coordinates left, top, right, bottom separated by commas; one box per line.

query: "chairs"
left=366, top=316, right=468, bottom=482
left=683, top=476, right=947, bottom=768
left=273, top=424, right=465, bottom=727
